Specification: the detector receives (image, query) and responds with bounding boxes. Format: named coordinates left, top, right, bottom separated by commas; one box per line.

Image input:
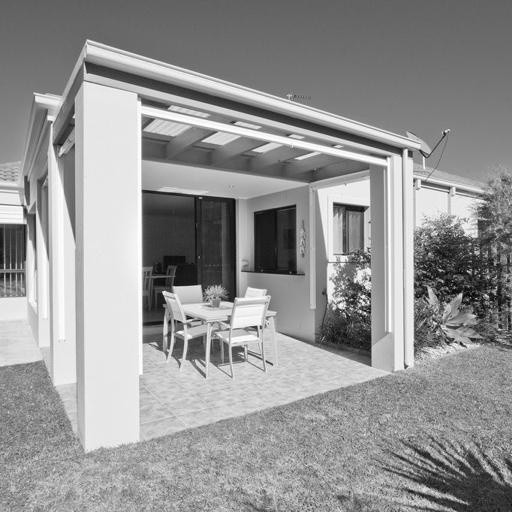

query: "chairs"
left=143, top=253, right=280, bottom=380
